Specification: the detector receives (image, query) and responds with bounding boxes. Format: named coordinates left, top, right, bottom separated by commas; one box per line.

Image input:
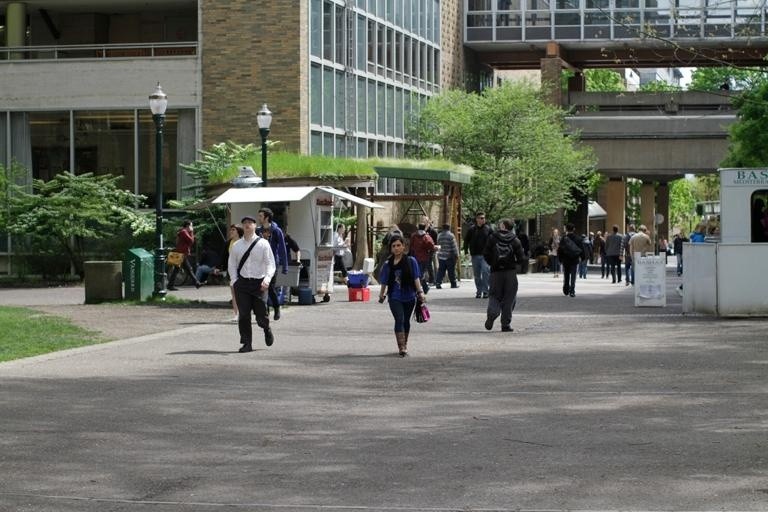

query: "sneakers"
left=429, top=281, right=441, bottom=289
left=602, top=276, right=634, bottom=285
left=563, top=291, right=575, bottom=297
left=167, top=286, right=178, bottom=291
left=502, top=325, right=513, bottom=332
left=231, top=306, right=281, bottom=352
left=676, top=286, right=683, bottom=296
left=196, top=280, right=207, bottom=289
left=477, top=292, right=488, bottom=298
left=486, top=320, right=493, bottom=329
left=451, top=285, right=459, bottom=288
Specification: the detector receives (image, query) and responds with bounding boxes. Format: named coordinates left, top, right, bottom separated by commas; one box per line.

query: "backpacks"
left=492, top=241, right=515, bottom=271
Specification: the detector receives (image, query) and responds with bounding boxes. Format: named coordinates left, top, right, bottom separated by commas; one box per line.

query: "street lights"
left=255, top=101, right=273, bottom=186
left=146, top=80, right=170, bottom=303
left=684, top=213, right=695, bottom=234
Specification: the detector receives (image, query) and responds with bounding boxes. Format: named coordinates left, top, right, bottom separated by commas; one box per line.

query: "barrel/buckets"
left=83, top=259, right=123, bottom=301
left=347, top=269, right=369, bottom=288
left=299, top=284, right=312, bottom=306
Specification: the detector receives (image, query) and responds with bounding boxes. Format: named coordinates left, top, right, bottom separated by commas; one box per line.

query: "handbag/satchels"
left=561, top=237, right=582, bottom=262
left=167, top=251, right=184, bottom=267
left=416, top=301, right=431, bottom=322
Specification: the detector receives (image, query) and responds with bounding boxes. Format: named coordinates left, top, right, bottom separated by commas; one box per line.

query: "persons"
left=373, top=212, right=721, bottom=355
left=167, top=208, right=349, bottom=352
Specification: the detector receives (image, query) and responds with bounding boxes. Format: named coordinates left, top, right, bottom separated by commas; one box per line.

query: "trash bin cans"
left=125, top=248, right=154, bottom=302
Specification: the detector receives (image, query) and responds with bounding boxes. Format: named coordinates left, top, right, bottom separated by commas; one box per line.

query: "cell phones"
left=379, top=295, right=386, bottom=303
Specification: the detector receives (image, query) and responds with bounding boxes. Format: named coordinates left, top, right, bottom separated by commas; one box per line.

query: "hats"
left=241, top=216, right=256, bottom=223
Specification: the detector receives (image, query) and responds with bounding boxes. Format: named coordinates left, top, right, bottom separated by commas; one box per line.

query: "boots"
left=396, top=332, right=408, bottom=357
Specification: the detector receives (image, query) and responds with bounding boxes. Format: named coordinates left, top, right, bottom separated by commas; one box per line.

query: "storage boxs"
left=274, top=264, right=301, bottom=287
